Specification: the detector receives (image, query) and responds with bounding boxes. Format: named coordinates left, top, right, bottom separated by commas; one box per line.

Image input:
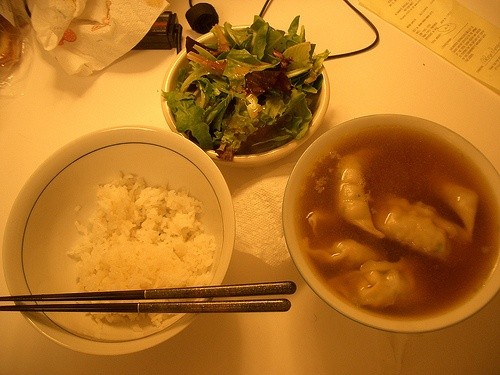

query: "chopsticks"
left=0, top=281, right=297, bottom=313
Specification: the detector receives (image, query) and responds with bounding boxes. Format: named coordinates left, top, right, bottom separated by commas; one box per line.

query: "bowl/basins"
left=282, top=114, right=500, bottom=333
left=2, top=124, right=236, bottom=355
left=160, top=25, right=331, bottom=166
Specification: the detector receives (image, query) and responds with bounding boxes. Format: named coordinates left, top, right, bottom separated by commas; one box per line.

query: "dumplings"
left=305, top=144, right=478, bottom=314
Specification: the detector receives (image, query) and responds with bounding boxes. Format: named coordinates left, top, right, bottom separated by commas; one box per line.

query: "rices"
left=64, top=173, right=213, bottom=327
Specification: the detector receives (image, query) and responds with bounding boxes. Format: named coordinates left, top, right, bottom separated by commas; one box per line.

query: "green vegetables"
left=162, top=13, right=330, bottom=159
